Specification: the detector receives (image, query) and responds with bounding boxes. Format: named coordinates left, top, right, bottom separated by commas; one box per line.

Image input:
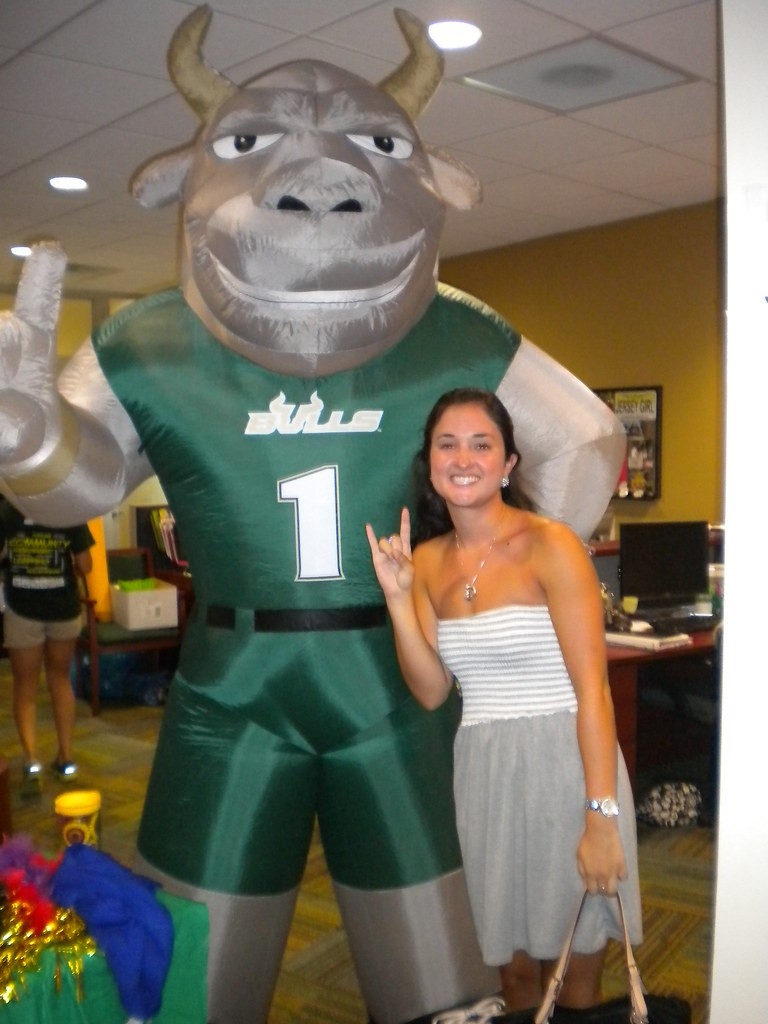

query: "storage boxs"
left=111, top=576, right=179, bottom=632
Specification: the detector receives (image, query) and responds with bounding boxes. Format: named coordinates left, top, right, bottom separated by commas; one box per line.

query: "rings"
left=387, top=536, right=393, bottom=543
left=599, top=886, right=606, bottom=890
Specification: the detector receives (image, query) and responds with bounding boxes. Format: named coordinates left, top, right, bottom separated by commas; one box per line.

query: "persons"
left=364, top=387, right=644, bottom=1012
left=0, top=492, right=95, bottom=794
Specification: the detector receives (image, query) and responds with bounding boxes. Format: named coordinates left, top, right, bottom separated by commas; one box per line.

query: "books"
left=604, top=629, right=692, bottom=651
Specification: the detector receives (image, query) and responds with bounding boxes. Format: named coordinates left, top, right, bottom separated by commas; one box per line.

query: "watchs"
left=585, top=797, right=620, bottom=818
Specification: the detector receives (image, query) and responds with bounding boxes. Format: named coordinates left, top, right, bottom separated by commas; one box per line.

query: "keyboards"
left=650, top=614, right=719, bottom=638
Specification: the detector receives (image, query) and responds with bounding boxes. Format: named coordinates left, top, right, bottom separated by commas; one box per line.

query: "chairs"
left=73, top=546, right=194, bottom=717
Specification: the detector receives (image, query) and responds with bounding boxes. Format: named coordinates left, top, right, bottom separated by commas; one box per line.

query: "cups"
left=55, top=790, right=100, bottom=850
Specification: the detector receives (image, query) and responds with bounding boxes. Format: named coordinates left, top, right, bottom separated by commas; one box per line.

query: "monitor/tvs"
left=618, top=521, right=710, bottom=611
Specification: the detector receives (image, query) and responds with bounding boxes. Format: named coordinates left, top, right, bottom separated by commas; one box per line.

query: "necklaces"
left=455, top=502, right=505, bottom=600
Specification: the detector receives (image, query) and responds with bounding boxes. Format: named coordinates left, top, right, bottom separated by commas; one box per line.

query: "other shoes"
left=21, top=764, right=39, bottom=780
left=48, top=761, right=76, bottom=780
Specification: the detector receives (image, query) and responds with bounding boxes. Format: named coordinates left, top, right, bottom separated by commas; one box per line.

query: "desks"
left=600, top=626, right=720, bottom=793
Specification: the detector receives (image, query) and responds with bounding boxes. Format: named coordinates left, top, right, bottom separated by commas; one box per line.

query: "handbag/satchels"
left=492, top=885, right=690, bottom=1024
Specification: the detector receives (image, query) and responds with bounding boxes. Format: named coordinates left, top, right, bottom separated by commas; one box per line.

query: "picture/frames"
left=587, top=386, right=663, bottom=499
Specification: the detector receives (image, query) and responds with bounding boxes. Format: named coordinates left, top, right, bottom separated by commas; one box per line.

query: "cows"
left=127, top=3, right=483, bottom=377
